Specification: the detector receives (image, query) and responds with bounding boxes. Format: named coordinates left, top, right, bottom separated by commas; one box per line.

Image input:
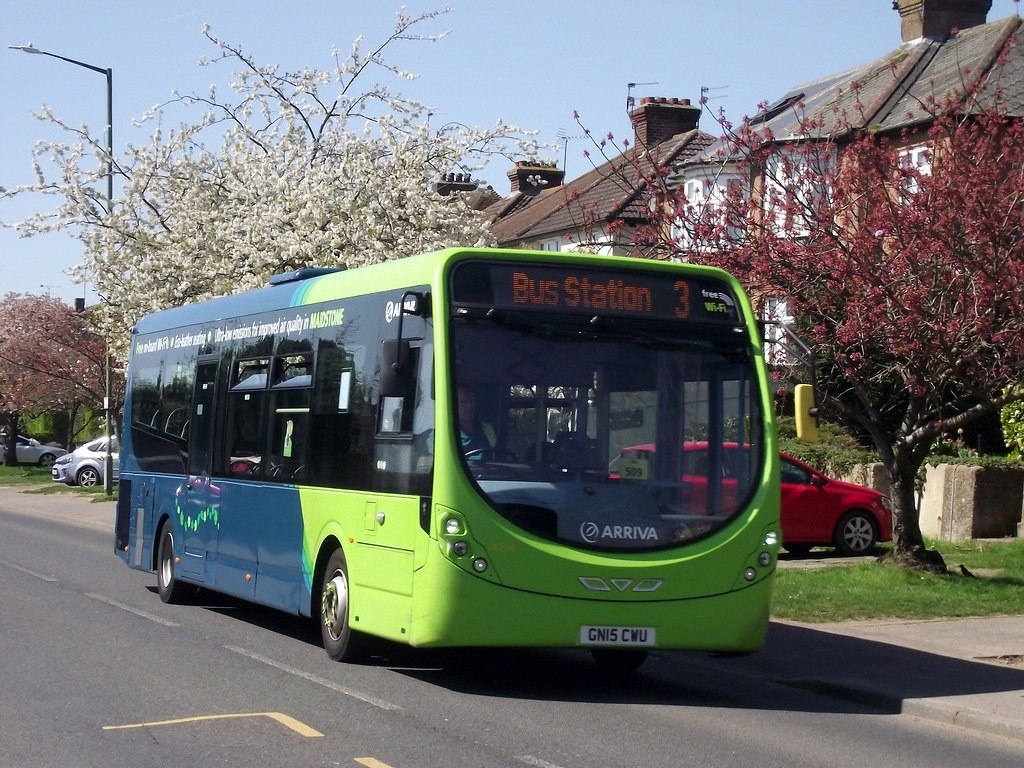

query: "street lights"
left=7, top=40, right=114, bottom=496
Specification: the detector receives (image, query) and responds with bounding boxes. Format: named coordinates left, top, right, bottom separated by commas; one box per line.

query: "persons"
left=427, top=382, right=493, bottom=462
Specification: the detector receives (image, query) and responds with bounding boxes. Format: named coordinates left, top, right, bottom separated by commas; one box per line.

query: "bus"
left=111, top=248, right=821, bottom=666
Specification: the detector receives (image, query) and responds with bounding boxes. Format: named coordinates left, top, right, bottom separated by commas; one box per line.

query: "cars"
left=51, top=433, right=123, bottom=488
left=0, top=432, right=68, bottom=468
left=608, top=441, right=894, bottom=557
